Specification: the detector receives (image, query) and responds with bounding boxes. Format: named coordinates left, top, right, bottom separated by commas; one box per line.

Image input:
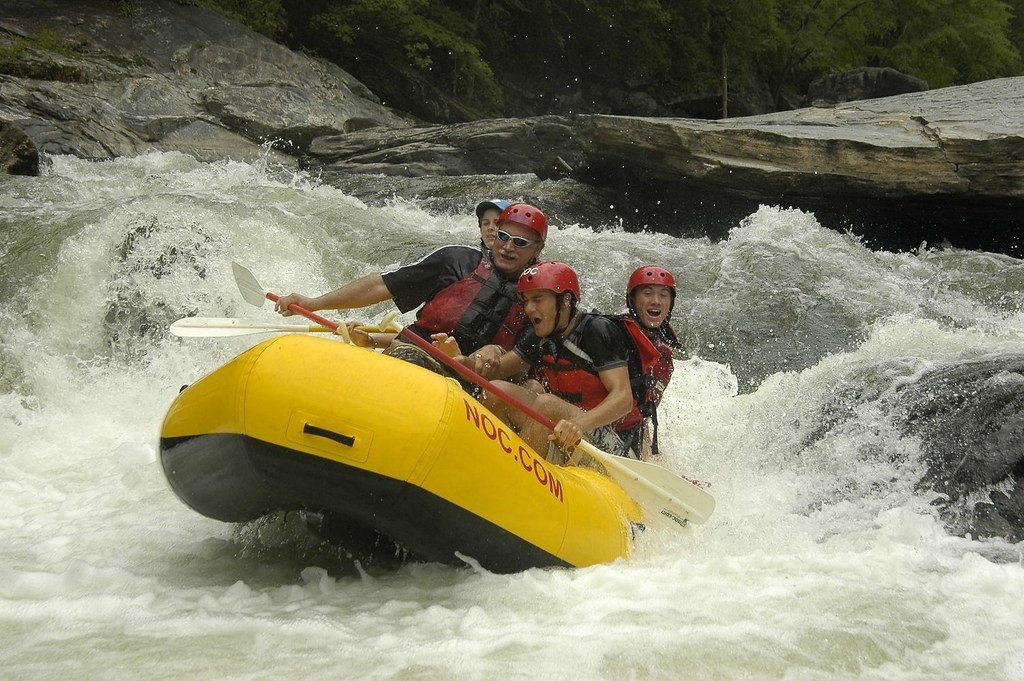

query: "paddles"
left=378, top=311, right=715, bottom=534
left=232, top=261, right=350, bottom=344
left=168, top=316, right=398, bottom=336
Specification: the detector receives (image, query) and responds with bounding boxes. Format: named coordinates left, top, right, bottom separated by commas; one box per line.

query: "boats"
left=158, top=330, right=701, bottom=576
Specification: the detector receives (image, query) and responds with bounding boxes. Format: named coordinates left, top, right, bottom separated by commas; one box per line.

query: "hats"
left=476, top=198, right=511, bottom=219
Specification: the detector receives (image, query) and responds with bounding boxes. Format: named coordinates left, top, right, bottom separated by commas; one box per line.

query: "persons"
left=274, top=203, right=548, bottom=391
left=431, top=261, right=633, bottom=459
left=612, top=266, right=681, bottom=457
left=338, top=200, right=512, bottom=349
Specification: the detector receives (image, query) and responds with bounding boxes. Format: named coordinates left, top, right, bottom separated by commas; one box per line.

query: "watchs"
left=497, top=345, right=505, bottom=355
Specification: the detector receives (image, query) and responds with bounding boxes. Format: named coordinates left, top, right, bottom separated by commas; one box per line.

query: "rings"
left=485, top=363, right=491, bottom=369
left=475, top=354, right=482, bottom=359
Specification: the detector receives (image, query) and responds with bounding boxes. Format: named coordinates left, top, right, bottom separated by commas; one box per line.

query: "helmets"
left=515, top=261, right=581, bottom=301
left=499, top=202, right=548, bottom=241
left=626, top=266, right=676, bottom=308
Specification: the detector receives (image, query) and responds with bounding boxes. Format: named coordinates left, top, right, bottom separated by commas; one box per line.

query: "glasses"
left=496, top=230, right=539, bottom=247
